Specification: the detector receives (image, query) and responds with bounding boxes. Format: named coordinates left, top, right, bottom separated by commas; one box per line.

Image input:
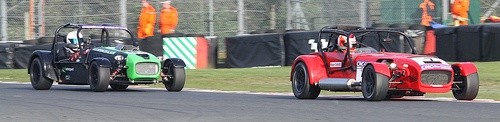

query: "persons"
left=136, top=0, right=156, bottom=42
left=332, top=32, right=357, bottom=53
left=58, top=30, right=89, bottom=61
left=449, top=0, right=470, bottom=26
left=158, top=1, right=178, bottom=36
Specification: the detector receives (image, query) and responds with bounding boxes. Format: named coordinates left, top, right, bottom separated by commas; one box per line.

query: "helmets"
left=66, top=31, right=84, bottom=46
left=338, top=33, right=356, bottom=50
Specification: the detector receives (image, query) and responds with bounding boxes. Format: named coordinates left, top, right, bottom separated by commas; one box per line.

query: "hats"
left=160, top=1, right=171, bottom=4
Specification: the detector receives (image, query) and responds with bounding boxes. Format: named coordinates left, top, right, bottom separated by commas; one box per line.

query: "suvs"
left=27, top=24, right=186, bottom=92
left=288, top=24, right=479, bottom=102
